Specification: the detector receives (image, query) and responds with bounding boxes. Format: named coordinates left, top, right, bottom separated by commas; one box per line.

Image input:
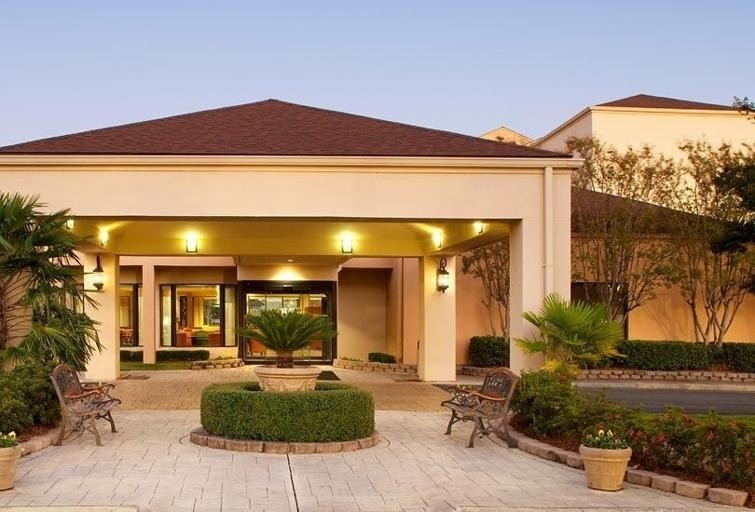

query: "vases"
left=0, top=446, right=21, bottom=489
left=579, top=444, right=633, bottom=491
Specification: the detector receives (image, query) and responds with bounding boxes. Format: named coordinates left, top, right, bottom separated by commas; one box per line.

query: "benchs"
left=439, top=367, right=522, bottom=449
left=47, top=364, right=121, bottom=447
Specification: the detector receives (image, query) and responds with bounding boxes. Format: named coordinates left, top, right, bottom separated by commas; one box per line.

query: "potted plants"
left=228, top=309, right=339, bottom=392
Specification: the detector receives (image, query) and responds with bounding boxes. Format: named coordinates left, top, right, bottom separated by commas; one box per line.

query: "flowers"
left=0, top=431, right=19, bottom=447
left=583, top=425, right=629, bottom=449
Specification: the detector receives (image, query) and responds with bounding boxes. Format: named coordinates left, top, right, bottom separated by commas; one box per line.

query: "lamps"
left=91, top=255, right=104, bottom=290
left=435, top=256, right=451, bottom=293
